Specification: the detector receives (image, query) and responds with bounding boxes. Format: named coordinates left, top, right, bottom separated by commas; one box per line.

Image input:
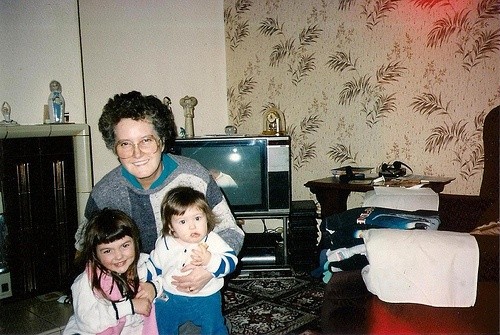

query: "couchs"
left=321, top=104, right=500, bottom=335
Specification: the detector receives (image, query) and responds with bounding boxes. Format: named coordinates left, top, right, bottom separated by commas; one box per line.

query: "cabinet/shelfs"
left=233, top=216, right=296, bottom=279
left=0, top=121, right=91, bottom=302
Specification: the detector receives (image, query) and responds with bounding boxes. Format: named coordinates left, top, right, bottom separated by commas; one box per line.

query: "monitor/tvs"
left=171, top=136, right=290, bottom=215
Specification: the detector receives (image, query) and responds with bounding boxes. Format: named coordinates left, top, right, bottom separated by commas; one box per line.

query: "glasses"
left=114, top=136, right=162, bottom=158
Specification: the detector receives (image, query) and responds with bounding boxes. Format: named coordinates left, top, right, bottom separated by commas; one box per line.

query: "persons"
left=147, top=187, right=238, bottom=335
left=84, top=91, right=245, bottom=257
left=63, top=206, right=164, bottom=335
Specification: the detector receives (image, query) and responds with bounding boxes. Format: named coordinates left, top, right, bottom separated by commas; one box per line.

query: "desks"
left=303, top=175, right=454, bottom=266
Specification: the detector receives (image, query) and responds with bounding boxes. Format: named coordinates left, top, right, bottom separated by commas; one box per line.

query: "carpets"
left=39, top=269, right=326, bottom=334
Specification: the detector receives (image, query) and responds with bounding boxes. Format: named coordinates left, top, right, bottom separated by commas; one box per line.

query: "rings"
left=189, top=286, right=193, bottom=292
